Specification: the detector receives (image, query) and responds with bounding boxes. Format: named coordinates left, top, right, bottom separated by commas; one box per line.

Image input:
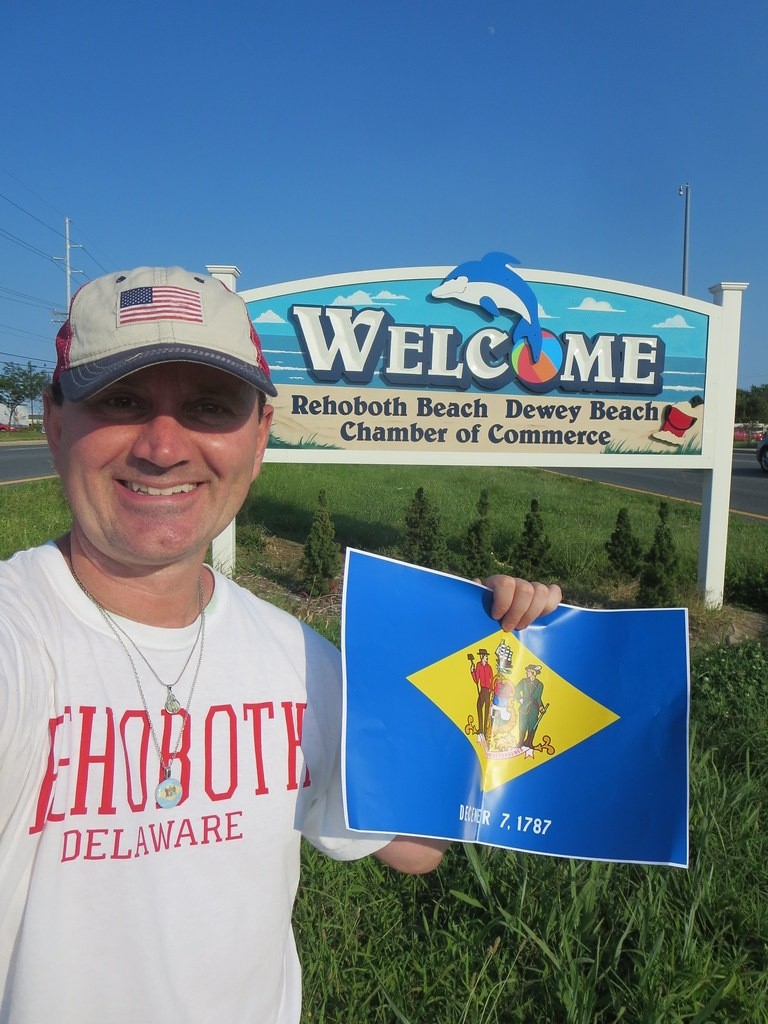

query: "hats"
left=53, top=267, right=277, bottom=402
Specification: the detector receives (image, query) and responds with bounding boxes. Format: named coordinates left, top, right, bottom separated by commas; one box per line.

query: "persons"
left=1, top=264, right=563, bottom=1024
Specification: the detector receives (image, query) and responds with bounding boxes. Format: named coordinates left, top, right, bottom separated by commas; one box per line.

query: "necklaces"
left=66, top=530, right=206, bottom=810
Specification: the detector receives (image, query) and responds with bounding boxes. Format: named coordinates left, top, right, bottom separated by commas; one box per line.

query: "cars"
left=734, top=424, right=768, bottom=470
left=0, top=423, right=16, bottom=432
